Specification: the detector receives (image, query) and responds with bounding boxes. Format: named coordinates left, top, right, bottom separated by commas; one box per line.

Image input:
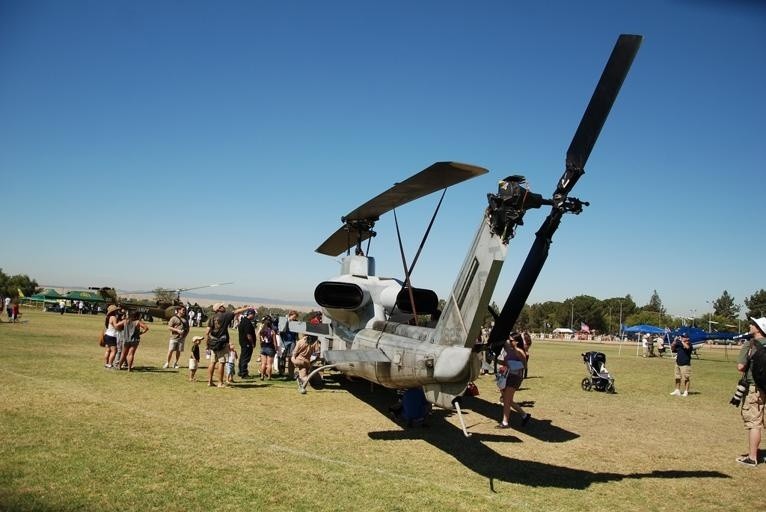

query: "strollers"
left=578, top=351, right=615, bottom=393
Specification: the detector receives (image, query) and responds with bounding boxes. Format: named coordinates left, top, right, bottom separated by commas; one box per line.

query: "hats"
left=191, top=336, right=203, bottom=342
left=750, top=317, right=766, bottom=334
left=106, top=305, right=120, bottom=316
left=212, top=303, right=224, bottom=312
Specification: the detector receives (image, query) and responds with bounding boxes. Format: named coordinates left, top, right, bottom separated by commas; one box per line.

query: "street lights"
left=617, top=299, right=625, bottom=337
left=569, top=300, right=576, bottom=330
left=689, top=309, right=697, bottom=328
left=607, top=304, right=613, bottom=334
left=490, top=318, right=494, bottom=329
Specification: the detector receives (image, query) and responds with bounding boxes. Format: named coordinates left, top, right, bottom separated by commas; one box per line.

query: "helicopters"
left=16, top=288, right=102, bottom=315
left=80, top=281, right=234, bottom=323
left=277, top=32, right=643, bottom=438
left=652, top=304, right=753, bottom=360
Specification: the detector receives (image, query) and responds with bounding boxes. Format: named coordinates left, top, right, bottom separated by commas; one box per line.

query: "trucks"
left=552, top=328, right=576, bottom=337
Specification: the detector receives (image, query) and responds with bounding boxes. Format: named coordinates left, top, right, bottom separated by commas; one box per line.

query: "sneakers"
left=496, top=423, right=508, bottom=429
left=681, top=391, right=688, bottom=397
left=521, top=413, right=531, bottom=427
left=669, top=390, right=680, bottom=396
left=736, top=457, right=757, bottom=467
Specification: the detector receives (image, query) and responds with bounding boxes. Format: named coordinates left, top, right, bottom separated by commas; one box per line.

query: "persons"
left=477, top=330, right=532, bottom=429
left=79, top=301, right=85, bottom=314
left=642, top=335, right=650, bottom=358
left=657, top=336, right=664, bottom=358
left=669, top=333, right=693, bottom=397
left=104, top=303, right=320, bottom=388
left=647, top=333, right=657, bottom=357
left=736, top=317, right=766, bottom=466
left=7, top=305, right=13, bottom=323
left=4, top=296, right=11, bottom=312
left=59, top=301, right=65, bottom=315
left=13, top=303, right=19, bottom=323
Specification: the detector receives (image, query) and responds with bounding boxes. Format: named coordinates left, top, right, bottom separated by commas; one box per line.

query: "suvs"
left=578, top=331, right=592, bottom=340
left=738, top=339, right=748, bottom=345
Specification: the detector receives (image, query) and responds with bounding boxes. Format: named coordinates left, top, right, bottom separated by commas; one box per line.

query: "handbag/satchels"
left=206, top=333, right=226, bottom=351
left=466, top=382, right=479, bottom=396
left=496, top=376, right=506, bottom=389
left=98, top=329, right=104, bottom=347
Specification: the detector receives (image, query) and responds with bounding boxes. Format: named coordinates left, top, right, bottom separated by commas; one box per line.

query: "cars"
left=710, top=338, right=737, bottom=346
left=622, top=335, right=641, bottom=342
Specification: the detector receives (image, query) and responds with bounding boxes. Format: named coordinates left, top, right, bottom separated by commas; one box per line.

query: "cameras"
left=729, top=381, right=749, bottom=408
left=183, top=325, right=187, bottom=332
left=676, top=337, right=680, bottom=341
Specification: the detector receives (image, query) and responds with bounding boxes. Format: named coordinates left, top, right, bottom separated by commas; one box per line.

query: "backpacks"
left=751, top=340, right=766, bottom=392
